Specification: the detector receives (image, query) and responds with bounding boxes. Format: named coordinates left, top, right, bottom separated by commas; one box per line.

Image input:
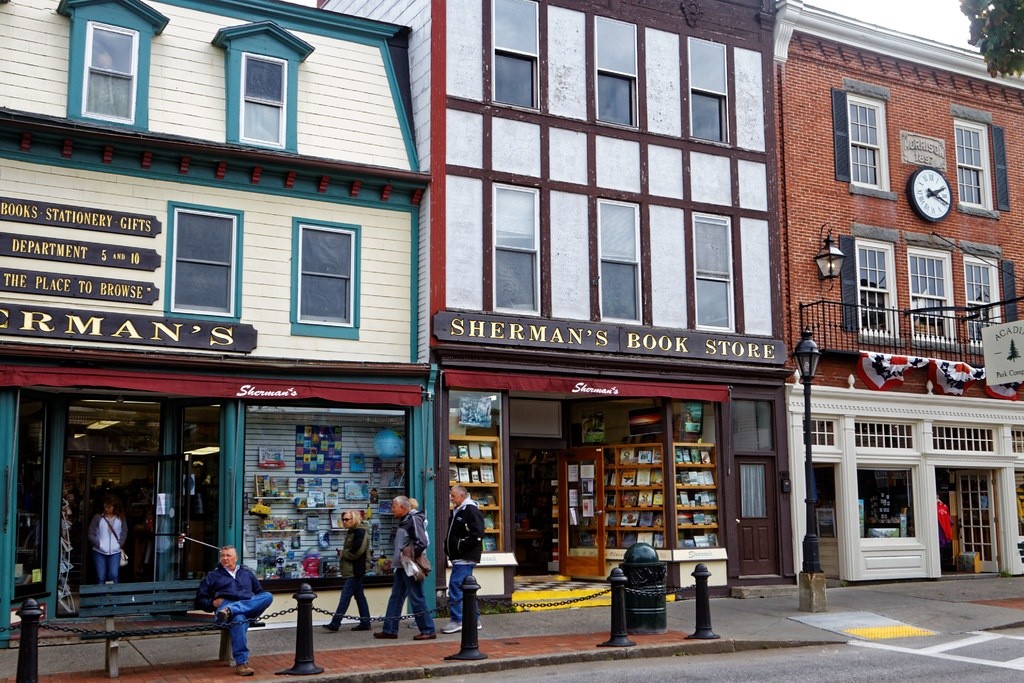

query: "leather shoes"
left=374, top=631, right=398, bottom=639
left=414, top=631, right=436, bottom=640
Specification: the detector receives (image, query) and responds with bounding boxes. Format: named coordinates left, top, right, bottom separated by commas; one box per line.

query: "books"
left=576, top=449, right=719, bottom=547
left=450, top=443, right=496, bottom=551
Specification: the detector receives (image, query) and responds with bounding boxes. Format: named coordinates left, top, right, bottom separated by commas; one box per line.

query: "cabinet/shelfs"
left=448, top=433, right=503, bottom=554
left=602, top=442, right=721, bottom=549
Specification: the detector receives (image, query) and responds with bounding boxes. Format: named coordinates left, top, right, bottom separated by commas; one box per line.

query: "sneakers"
left=476, top=618, right=483, bottom=630
left=440, top=621, right=462, bottom=634
left=234, top=663, right=255, bottom=676
left=408, top=621, right=420, bottom=629
left=217, top=607, right=231, bottom=623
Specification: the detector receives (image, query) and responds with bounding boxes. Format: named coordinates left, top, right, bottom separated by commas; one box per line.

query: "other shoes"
left=351, top=623, right=372, bottom=631
left=322, top=623, right=339, bottom=632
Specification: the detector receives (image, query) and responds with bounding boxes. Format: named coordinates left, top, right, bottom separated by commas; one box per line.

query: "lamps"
left=812, top=222, right=844, bottom=291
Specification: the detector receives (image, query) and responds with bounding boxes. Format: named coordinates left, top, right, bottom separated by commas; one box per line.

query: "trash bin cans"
left=619, top=543, right=668, bottom=635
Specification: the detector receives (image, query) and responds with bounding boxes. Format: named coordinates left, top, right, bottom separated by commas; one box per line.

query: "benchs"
left=78, top=580, right=266, bottom=678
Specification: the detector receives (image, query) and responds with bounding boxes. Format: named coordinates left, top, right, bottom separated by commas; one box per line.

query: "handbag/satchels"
left=119, top=549, right=128, bottom=567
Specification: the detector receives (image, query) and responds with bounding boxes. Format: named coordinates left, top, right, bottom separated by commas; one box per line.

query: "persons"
left=441, top=484, right=485, bottom=633
left=373, top=496, right=437, bottom=640
left=322, top=509, right=372, bottom=631
left=193, top=545, right=273, bottom=676
left=461, top=399, right=491, bottom=424
left=88, top=495, right=127, bottom=584
left=1017, top=484, right=1024, bottom=524
left=542, top=492, right=556, bottom=528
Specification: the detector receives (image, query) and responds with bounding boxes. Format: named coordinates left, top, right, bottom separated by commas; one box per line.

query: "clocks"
left=907, top=165, right=953, bottom=222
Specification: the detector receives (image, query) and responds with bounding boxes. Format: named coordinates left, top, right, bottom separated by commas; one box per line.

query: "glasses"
left=342, top=517, right=353, bottom=522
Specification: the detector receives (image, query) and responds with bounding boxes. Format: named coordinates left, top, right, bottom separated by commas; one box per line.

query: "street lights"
left=789, top=322, right=828, bottom=615
